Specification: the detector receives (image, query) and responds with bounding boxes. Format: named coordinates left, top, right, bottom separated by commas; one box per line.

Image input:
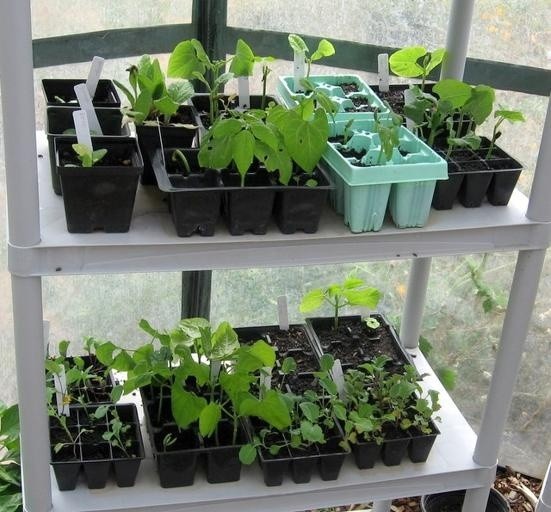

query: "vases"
left=421, top=482, right=512, bottom=511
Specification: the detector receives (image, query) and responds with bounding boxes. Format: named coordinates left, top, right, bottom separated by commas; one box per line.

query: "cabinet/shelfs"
left=0, top=0, right=551, bottom=510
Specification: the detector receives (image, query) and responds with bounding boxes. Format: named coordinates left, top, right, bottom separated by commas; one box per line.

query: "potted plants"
left=299, top=279, right=441, bottom=471
left=114, top=34, right=338, bottom=237
left=219, top=323, right=351, bottom=487
left=94, top=315, right=291, bottom=488
left=30, top=334, right=147, bottom=490
left=366, top=44, right=526, bottom=210
left=277, top=33, right=450, bottom=233
left=41, top=55, right=145, bottom=232
